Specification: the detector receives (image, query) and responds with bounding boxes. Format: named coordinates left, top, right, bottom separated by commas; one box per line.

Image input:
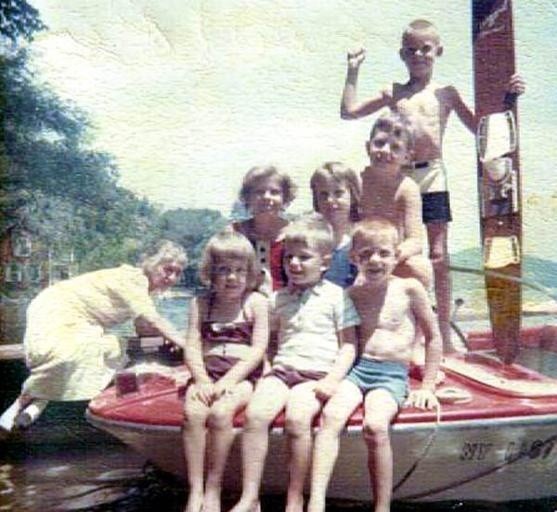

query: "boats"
left=85, top=320, right=557, bottom=504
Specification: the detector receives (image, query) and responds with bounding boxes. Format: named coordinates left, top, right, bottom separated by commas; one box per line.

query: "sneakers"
left=0, top=399, right=49, bottom=433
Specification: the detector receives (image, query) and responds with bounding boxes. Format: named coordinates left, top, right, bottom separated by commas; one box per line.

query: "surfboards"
left=472, top=0, right=522, bottom=365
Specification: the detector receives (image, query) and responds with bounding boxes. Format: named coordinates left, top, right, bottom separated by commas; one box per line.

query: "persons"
left=350, top=110, right=446, bottom=384
left=310, top=161, right=366, bottom=292
left=0, top=241, right=185, bottom=433
left=340, top=18, right=529, bottom=356
left=222, top=162, right=297, bottom=360
left=222, top=210, right=363, bottom=512
left=180, top=229, right=273, bottom=512
left=307, top=216, right=444, bottom=512
